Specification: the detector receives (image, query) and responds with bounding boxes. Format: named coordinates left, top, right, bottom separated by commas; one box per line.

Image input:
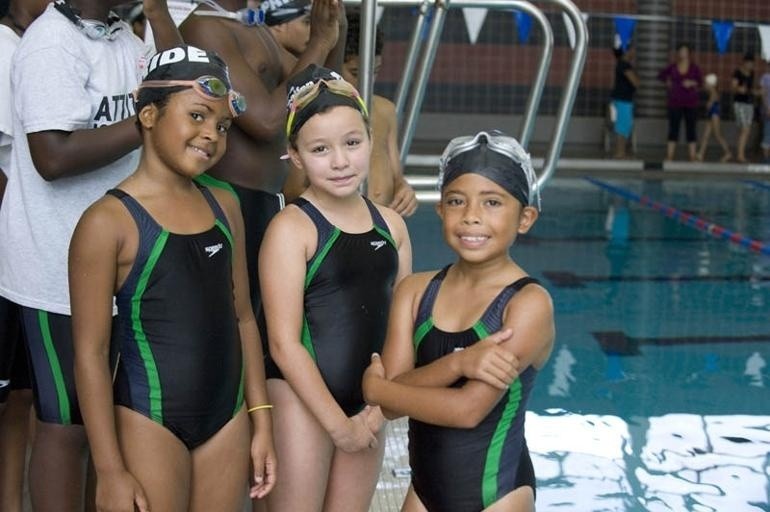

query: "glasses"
left=55, top=0, right=124, bottom=42
left=193, top=8, right=265, bottom=27
left=285, top=77, right=368, bottom=141
left=134, top=73, right=249, bottom=118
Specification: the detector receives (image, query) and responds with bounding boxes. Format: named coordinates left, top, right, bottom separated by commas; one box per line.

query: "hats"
left=136, top=44, right=230, bottom=109
left=705, top=72, right=717, bottom=87
left=261, top=0, right=315, bottom=27
left=287, top=64, right=368, bottom=143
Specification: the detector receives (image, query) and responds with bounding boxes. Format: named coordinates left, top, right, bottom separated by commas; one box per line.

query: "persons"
left=760, top=60, right=770, bottom=164
left=611, top=34, right=640, bottom=160
left=253, top=69, right=415, bottom=511
left=659, top=40, right=707, bottom=162
left=2, top=0, right=349, bottom=512
left=284, top=15, right=418, bottom=220
left=604, top=79, right=639, bottom=158
left=695, top=72, right=734, bottom=163
left=729, top=51, right=765, bottom=162
left=359, top=130, right=556, bottom=511
